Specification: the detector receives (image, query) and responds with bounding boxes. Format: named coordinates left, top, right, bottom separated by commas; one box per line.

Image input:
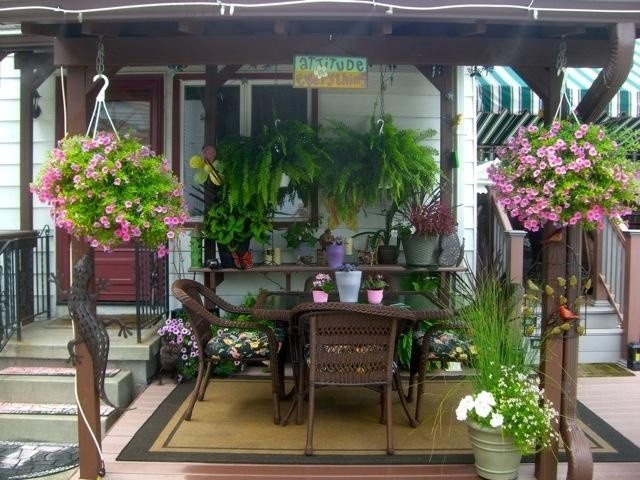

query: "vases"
left=367, top=289, right=383, bottom=304
left=312, top=290, right=328, bottom=303
left=464, top=415, right=523, bottom=480
left=399, top=233, right=439, bottom=267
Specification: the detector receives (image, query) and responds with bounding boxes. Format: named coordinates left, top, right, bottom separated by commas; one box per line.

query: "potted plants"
left=351, top=191, right=424, bottom=264
left=198, top=184, right=274, bottom=269
left=211, top=117, right=335, bottom=212
left=281, top=213, right=325, bottom=265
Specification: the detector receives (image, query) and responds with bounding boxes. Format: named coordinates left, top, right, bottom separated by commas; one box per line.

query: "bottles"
left=273, top=248, right=281, bottom=266
left=190, top=223, right=202, bottom=268
left=264, top=247, right=272, bottom=266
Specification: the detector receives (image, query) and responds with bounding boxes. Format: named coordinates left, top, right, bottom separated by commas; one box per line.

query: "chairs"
left=408, top=283, right=526, bottom=423
left=288, top=300, right=418, bottom=457
left=172, top=278, right=292, bottom=425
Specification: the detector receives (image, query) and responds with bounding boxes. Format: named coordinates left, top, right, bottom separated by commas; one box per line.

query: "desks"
left=253, top=289, right=451, bottom=428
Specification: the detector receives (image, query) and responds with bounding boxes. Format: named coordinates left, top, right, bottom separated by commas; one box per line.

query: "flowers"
left=308, top=272, right=336, bottom=295
left=360, top=272, right=389, bottom=290
left=404, top=265, right=588, bottom=476
left=382, top=179, right=462, bottom=240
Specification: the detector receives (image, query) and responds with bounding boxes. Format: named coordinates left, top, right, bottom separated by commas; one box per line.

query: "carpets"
left=42, top=312, right=162, bottom=329
left=0, top=365, right=122, bottom=480
left=530, top=363, right=636, bottom=381
left=113, top=373, right=639, bottom=465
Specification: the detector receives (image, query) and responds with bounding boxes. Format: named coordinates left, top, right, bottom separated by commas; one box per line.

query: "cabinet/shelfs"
left=187, top=262, right=470, bottom=312
